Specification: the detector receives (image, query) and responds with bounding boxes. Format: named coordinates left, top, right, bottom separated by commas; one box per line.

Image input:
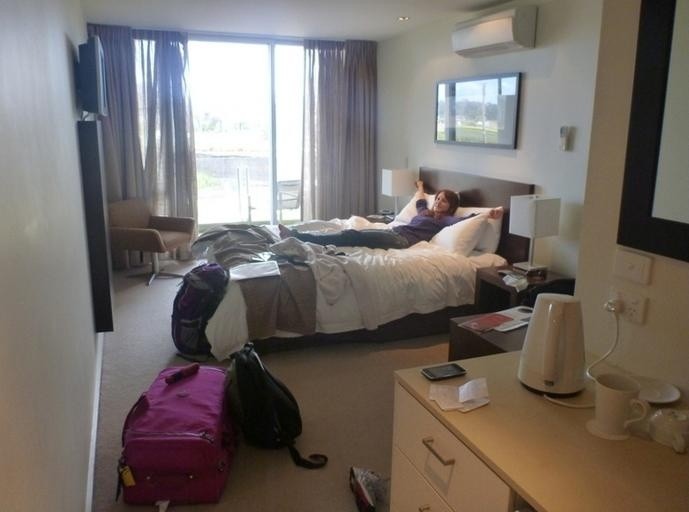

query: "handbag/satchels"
left=117, top=365, right=237, bottom=504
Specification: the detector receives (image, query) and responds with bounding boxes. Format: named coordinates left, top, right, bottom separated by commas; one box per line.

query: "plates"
left=627, top=375, right=681, bottom=406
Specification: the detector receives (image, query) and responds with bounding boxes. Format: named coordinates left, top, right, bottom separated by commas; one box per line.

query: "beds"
left=188, top=160, right=536, bottom=361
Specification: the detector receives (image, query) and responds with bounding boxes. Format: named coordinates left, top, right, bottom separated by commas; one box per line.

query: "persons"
left=278, top=180, right=505, bottom=249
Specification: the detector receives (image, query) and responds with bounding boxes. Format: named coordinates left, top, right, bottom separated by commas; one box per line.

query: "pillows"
left=393, top=189, right=461, bottom=225
left=427, top=213, right=489, bottom=258
left=453, top=206, right=504, bottom=255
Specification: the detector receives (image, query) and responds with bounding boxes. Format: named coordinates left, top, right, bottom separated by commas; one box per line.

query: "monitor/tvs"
left=80, top=36, right=109, bottom=117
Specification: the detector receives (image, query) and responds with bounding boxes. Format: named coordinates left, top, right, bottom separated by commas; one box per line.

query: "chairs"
left=105, top=197, right=196, bottom=287
left=273, top=179, right=305, bottom=224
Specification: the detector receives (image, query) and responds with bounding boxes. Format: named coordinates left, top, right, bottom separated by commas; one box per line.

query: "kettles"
left=518, top=292, right=587, bottom=396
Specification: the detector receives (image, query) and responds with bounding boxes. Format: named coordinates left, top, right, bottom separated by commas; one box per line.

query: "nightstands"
left=475, top=262, right=573, bottom=311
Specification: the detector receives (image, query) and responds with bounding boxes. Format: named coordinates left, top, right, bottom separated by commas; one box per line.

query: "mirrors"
left=618, top=0, right=689, bottom=261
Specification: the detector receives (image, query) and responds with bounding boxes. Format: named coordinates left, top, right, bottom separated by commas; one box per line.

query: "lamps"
left=506, top=194, right=561, bottom=276
left=379, top=167, right=417, bottom=217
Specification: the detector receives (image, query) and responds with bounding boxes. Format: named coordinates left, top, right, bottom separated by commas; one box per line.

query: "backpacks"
left=224, top=340, right=301, bottom=450
left=171, top=262, right=230, bottom=354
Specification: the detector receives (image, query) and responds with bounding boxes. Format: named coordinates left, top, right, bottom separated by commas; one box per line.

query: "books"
left=458, top=314, right=513, bottom=335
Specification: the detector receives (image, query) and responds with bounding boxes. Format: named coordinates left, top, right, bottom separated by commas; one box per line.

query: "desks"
left=389, top=348, right=688, bottom=512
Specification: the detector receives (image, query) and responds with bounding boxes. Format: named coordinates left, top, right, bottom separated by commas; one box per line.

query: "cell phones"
left=421, top=363, right=466, bottom=380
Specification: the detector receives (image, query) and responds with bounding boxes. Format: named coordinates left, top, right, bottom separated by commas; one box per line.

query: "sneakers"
left=349, top=466, right=381, bottom=512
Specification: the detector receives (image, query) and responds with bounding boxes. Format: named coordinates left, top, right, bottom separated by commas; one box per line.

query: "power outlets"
left=601, top=287, right=649, bottom=323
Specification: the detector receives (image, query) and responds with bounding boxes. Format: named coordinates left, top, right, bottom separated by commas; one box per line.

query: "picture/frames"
left=432, top=70, right=523, bottom=149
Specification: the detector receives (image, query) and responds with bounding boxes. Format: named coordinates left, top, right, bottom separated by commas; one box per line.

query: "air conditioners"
left=448, top=3, right=540, bottom=56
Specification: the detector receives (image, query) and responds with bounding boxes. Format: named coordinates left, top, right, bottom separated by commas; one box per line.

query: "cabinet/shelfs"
left=448, top=312, right=530, bottom=360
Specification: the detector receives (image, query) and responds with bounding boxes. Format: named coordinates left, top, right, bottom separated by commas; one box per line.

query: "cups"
left=593, top=371, right=650, bottom=435
left=648, top=409, right=688, bottom=451
